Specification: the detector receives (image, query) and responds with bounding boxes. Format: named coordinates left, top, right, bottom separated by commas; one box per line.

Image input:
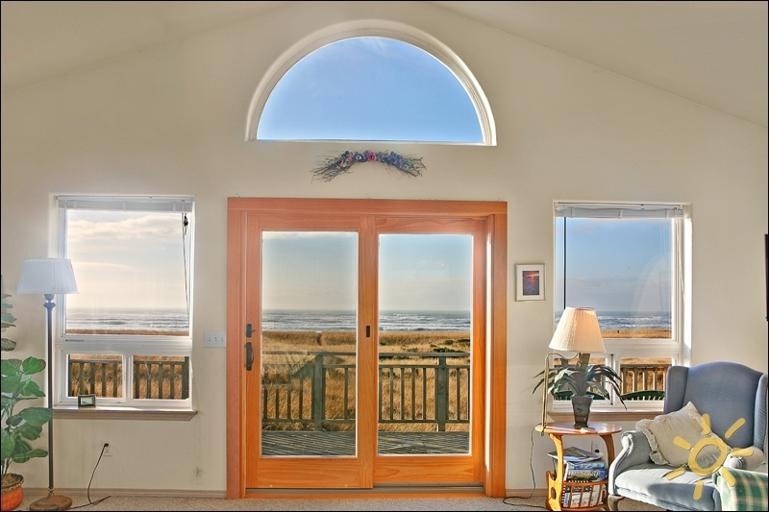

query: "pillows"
left=635, top=401, right=732, bottom=467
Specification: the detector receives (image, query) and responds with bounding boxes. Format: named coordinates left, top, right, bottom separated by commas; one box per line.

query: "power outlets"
left=592, top=441, right=604, bottom=458
left=102, top=440, right=112, bottom=457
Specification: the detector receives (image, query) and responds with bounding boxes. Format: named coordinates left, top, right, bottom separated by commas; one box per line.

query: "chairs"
left=712, top=468, right=768, bottom=511
left=607, top=361, right=768, bottom=512
left=621, top=391, right=665, bottom=401
left=556, top=390, right=605, bottom=400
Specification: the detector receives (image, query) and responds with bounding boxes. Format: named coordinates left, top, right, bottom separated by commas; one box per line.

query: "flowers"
left=310, top=149, right=426, bottom=183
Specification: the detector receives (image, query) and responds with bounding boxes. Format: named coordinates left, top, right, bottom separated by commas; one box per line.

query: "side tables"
left=535, top=423, right=622, bottom=512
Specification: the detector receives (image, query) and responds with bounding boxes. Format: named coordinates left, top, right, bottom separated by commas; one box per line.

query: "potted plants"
left=1, top=295, right=54, bottom=511
left=533, top=364, right=627, bottom=430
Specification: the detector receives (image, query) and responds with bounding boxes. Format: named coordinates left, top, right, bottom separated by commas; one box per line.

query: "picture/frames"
left=515, top=263, right=545, bottom=301
left=78, top=395, right=96, bottom=408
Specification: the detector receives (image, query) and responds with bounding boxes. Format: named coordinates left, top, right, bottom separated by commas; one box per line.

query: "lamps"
left=17, top=258, right=78, bottom=512
left=540, top=306, right=605, bottom=436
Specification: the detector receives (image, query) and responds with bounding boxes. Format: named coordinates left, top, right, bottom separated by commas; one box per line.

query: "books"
left=547, top=446, right=605, bottom=509
